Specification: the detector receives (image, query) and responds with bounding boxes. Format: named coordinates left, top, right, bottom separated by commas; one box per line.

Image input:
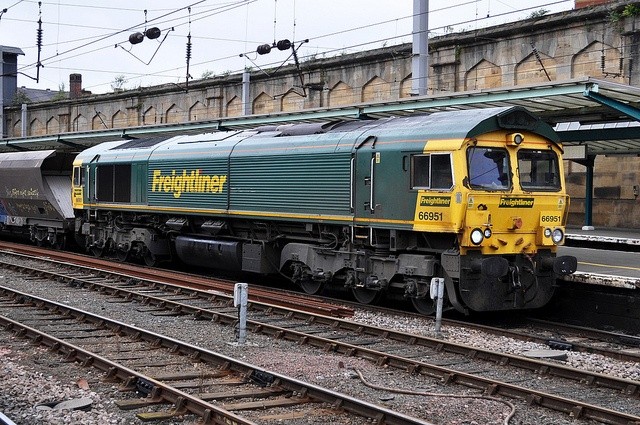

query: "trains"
left=0, top=106, right=577, bottom=315
